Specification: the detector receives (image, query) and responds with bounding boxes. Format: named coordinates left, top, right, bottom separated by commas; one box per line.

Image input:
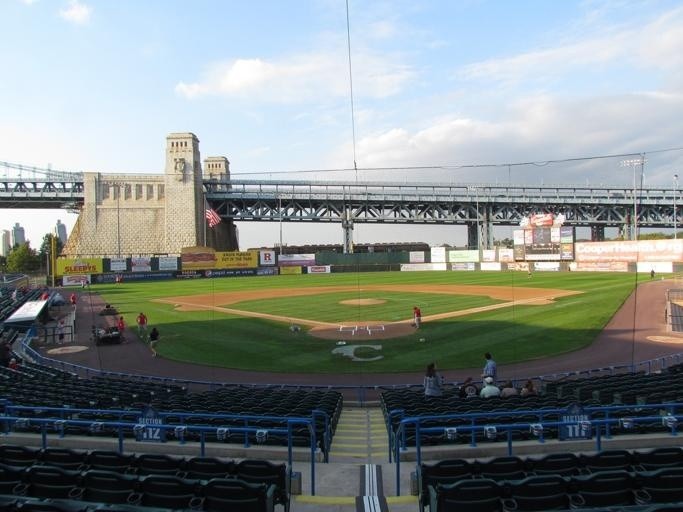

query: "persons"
left=413, top=306, right=421, bottom=329
left=118, top=311, right=161, bottom=358
left=424, top=353, right=541, bottom=400
left=70, top=293, right=76, bottom=309
left=41, top=291, right=49, bottom=301
left=0, top=337, right=17, bottom=369
left=650, top=269, right=655, bottom=280
left=57, top=319, right=65, bottom=345
left=528, top=272, right=533, bottom=281
left=0, top=285, right=25, bottom=302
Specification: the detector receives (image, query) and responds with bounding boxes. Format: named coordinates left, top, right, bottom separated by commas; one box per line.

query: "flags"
left=205, top=198, right=221, bottom=230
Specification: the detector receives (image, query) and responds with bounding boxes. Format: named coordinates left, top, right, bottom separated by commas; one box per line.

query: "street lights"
left=44, top=241, right=50, bottom=278
left=464, top=184, right=483, bottom=262
left=279, top=195, right=282, bottom=255
left=109, top=181, right=126, bottom=258
left=674, top=175, right=678, bottom=239
left=624, top=160, right=648, bottom=239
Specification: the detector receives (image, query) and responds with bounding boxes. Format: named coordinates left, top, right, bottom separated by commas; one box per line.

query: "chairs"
left=0, top=352, right=344, bottom=512
left=0, top=285, right=51, bottom=344
left=380, top=363, right=683, bottom=512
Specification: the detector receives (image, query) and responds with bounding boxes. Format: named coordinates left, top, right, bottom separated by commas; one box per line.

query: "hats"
left=484, top=377, right=494, bottom=384
